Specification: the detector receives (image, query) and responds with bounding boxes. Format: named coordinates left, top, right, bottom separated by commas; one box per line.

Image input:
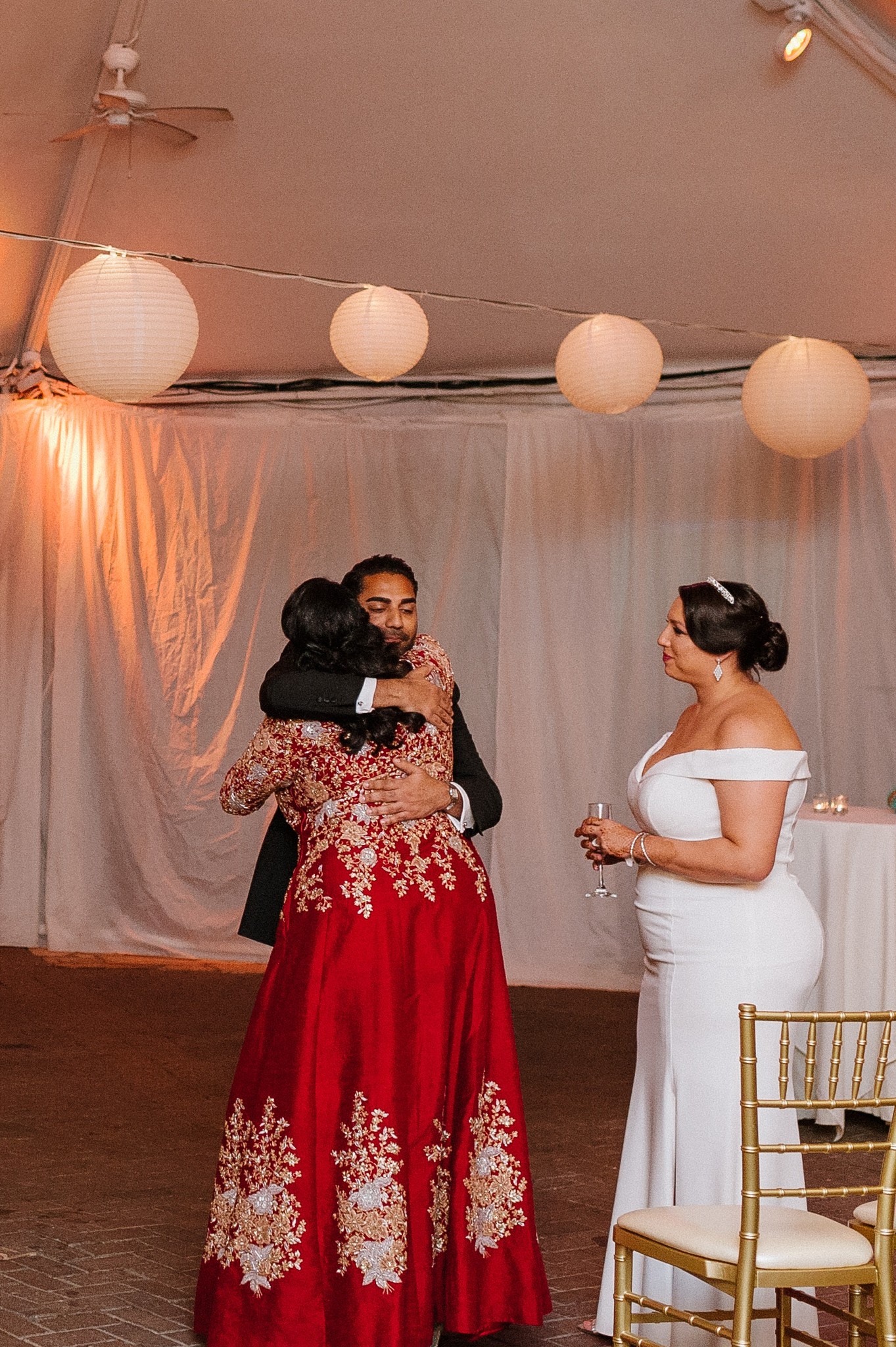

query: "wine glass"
left=584, top=803, right=617, bottom=898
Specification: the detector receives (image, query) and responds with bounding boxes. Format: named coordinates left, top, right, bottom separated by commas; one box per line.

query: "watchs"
left=442, top=779, right=461, bottom=813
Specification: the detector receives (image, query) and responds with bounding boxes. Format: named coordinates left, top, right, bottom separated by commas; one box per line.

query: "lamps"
left=46, top=250, right=200, bottom=405
left=328, top=285, right=431, bottom=382
left=741, top=337, right=871, bottom=460
left=555, top=312, right=664, bottom=415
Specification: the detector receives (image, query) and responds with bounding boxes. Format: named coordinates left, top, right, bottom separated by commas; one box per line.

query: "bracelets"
left=641, top=831, right=660, bottom=867
left=630, top=831, right=648, bottom=866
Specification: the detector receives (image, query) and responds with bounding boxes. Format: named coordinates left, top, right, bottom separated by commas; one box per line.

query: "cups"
left=833, top=797, right=848, bottom=815
left=813, top=793, right=829, bottom=813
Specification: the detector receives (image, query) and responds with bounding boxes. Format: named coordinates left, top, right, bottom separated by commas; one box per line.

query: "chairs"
left=610, top=1001, right=896, bottom=1347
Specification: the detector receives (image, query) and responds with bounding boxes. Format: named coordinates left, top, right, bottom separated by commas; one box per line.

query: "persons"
left=572, top=577, right=830, bottom=1347
left=235, top=554, right=506, bottom=952
left=193, top=577, right=555, bottom=1347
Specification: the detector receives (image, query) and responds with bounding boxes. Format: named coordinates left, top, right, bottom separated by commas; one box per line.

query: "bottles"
left=888, top=785, right=896, bottom=808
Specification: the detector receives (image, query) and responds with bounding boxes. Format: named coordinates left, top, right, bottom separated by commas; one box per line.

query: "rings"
left=591, top=837, right=600, bottom=847
